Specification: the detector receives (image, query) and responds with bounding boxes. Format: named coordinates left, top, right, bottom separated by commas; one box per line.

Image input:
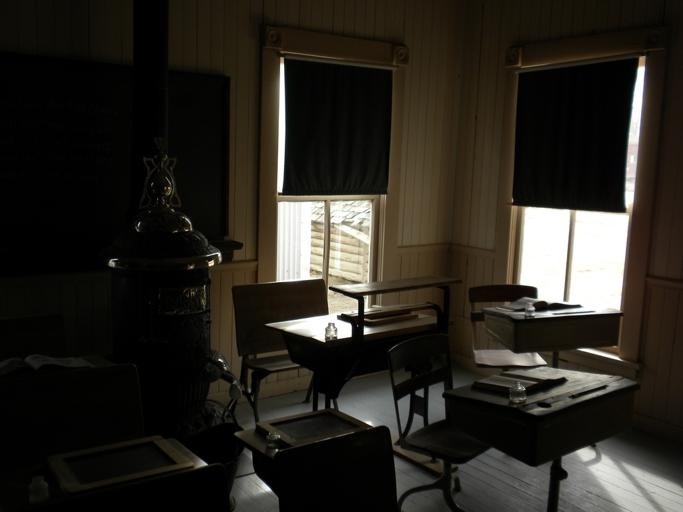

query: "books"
left=475, top=368, right=567, bottom=394
left=502, top=297, right=580, bottom=311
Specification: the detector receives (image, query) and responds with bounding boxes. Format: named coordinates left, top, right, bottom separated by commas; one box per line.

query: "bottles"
left=326, top=322, right=337, bottom=338
left=526, top=302, right=534, bottom=317
left=510, top=380, right=528, bottom=403
left=266, top=432, right=280, bottom=448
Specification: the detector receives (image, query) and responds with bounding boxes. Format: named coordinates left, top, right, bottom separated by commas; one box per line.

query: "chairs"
left=230, top=280, right=338, bottom=425
left=467, top=285, right=538, bottom=375
left=0, top=278, right=548, bottom=511
left=382, top=333, right=495, bottom=511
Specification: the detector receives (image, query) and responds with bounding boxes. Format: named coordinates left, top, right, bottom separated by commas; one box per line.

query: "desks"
left=231, top=407, right=401, bottom=510
left=441, top=365, right=641, bottom=512
left=481, top=303, right=623, bottom=448
left=6, top=436, right=229, bottom=509
left=263, top=277, right=463, bottom=462
